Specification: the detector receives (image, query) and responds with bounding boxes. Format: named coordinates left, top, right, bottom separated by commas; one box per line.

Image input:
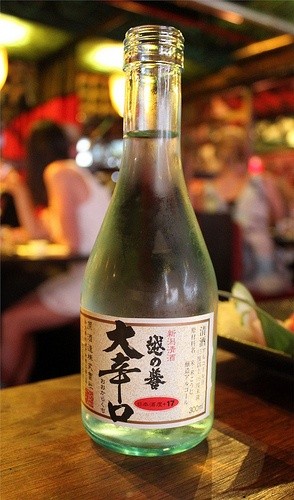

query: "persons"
left=182, top=140, right=294, bottom=301
left=0, top=119, right=111, bottom=387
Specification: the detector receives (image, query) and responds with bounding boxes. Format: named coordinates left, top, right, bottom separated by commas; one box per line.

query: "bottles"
left=230, top=155, right=288, bottom=299
left=80, top=24, right=216, bottom=457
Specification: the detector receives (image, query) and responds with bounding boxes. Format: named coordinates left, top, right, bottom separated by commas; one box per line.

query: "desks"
left=0, top=252, right=91, bottom=276
left=1, top=348, right=294, bottom=500
